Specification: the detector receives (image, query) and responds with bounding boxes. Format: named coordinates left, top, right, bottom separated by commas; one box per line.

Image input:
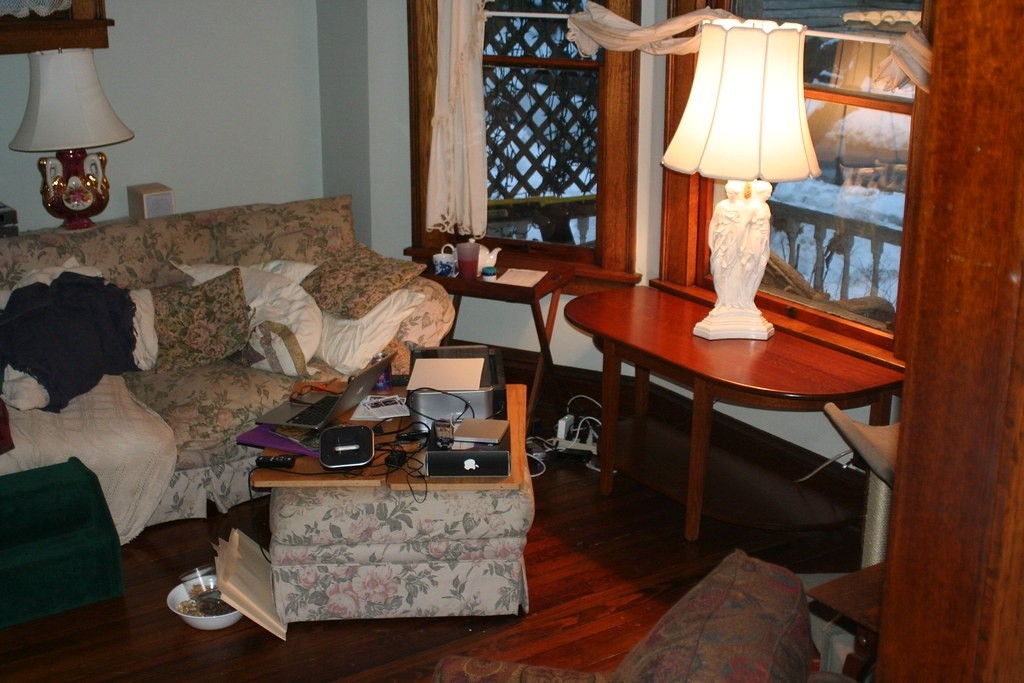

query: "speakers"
left=127, top=182, right=174, bottom=220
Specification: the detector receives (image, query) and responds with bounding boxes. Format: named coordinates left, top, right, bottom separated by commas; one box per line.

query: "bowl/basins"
left=167, top=575, right=243, bottom=631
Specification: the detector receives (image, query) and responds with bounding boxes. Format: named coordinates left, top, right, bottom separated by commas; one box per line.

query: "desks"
left=564, top=285, right=905, bottom=542
left=420, top=264, right=576, bottom=436
left=251, top=383, right=534, bottom=624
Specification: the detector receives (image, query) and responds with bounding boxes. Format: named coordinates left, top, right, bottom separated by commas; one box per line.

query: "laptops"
left=254, top=349, right=398, bottom=430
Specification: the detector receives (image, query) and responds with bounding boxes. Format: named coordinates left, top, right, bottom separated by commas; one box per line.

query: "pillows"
left=129, top=242, right=427, bottom=375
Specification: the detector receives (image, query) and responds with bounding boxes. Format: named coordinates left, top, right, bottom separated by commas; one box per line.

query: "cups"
left=433, top=253, right=459, bottom=278
left=178, top=563, right=226, bottom=617
left=457, top=243, right=480, bottom=281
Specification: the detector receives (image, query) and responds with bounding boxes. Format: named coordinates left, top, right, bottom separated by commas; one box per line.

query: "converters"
left=386, top=450, right=407, bottom=468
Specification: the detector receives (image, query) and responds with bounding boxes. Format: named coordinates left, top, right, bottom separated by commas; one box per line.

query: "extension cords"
left=554, top=438, right=599, bottom=457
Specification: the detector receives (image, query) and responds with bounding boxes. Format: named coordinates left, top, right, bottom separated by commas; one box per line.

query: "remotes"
left=257, top=455, right=294, bottom=468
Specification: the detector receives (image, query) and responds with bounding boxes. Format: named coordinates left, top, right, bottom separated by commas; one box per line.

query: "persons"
left=708, top=181, right=773, bottom=311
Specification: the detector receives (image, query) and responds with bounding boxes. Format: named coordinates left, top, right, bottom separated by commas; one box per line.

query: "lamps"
left=659, top=19, right=822, bottom=340
left=9, top=48, right=134, bottom=230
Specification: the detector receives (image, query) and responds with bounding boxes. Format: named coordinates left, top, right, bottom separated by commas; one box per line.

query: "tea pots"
left=441, top=238, right=502, bottom=277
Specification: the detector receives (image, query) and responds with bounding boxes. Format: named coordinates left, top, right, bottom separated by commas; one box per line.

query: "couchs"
left=433, top=552, right=813, bottom=683
left=1, top=194, right=456, bottom=527
